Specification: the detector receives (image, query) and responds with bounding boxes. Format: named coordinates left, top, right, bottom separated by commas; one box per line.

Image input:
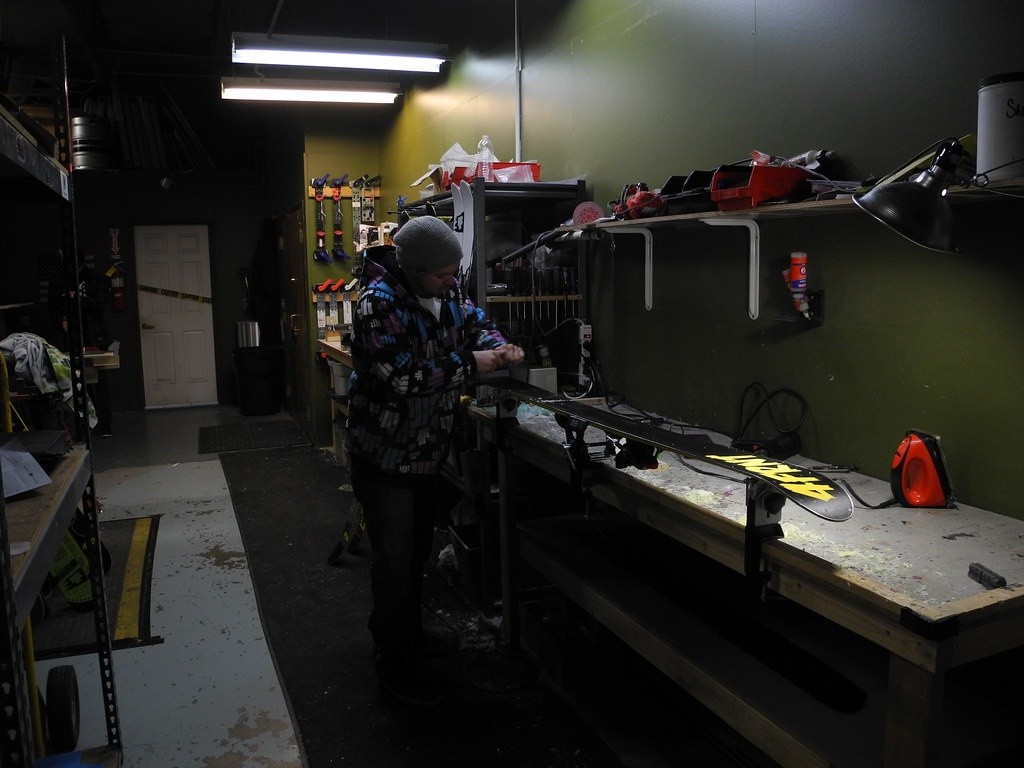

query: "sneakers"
left=376, top=652, right=446, bottom=708
left=372, top=626, right=446, bottom=651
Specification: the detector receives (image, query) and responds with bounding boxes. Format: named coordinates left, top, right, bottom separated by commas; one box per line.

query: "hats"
left=392, top=215, right=463, bottom=282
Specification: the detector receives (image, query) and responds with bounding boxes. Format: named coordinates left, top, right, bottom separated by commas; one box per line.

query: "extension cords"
left=577, top=325, right=593, bottom=386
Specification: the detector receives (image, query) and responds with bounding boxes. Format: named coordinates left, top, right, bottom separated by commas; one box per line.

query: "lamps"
left=220, top=76, right=404, bottom=104
left=229, top=31, right=455, bottom=73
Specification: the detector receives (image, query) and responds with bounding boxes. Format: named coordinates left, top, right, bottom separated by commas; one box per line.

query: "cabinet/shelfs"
left=395, top=174, right=588, bottom=376
left=1, top=0, right=124, bottom=768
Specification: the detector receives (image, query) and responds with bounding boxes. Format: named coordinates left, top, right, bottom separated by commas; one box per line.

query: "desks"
left=320, top=337, right=1024, bottom=768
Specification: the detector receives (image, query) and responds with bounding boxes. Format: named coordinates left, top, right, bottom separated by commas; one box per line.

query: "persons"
left=348, top=216, right=525, bottom=706
